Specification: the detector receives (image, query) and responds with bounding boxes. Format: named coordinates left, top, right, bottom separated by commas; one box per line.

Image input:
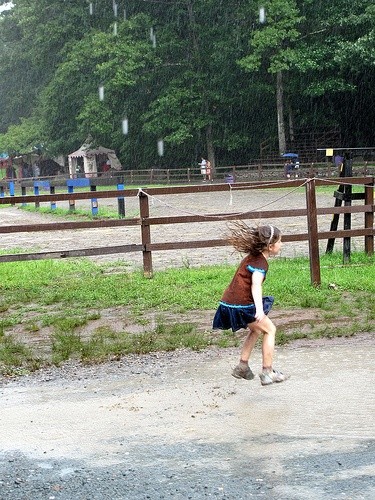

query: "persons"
left=212, top=220, right=291, bottom=386
left=198, top=157, right=211, bottom=183
left=100, top=156, right=111, bottom=172
left=284, top=158, right=294, bottom=179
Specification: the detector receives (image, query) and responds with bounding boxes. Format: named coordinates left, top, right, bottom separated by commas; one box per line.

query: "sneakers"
left=260, top=370, right=291, bottom=385
left=231, top=366, right=255, bottom=380
